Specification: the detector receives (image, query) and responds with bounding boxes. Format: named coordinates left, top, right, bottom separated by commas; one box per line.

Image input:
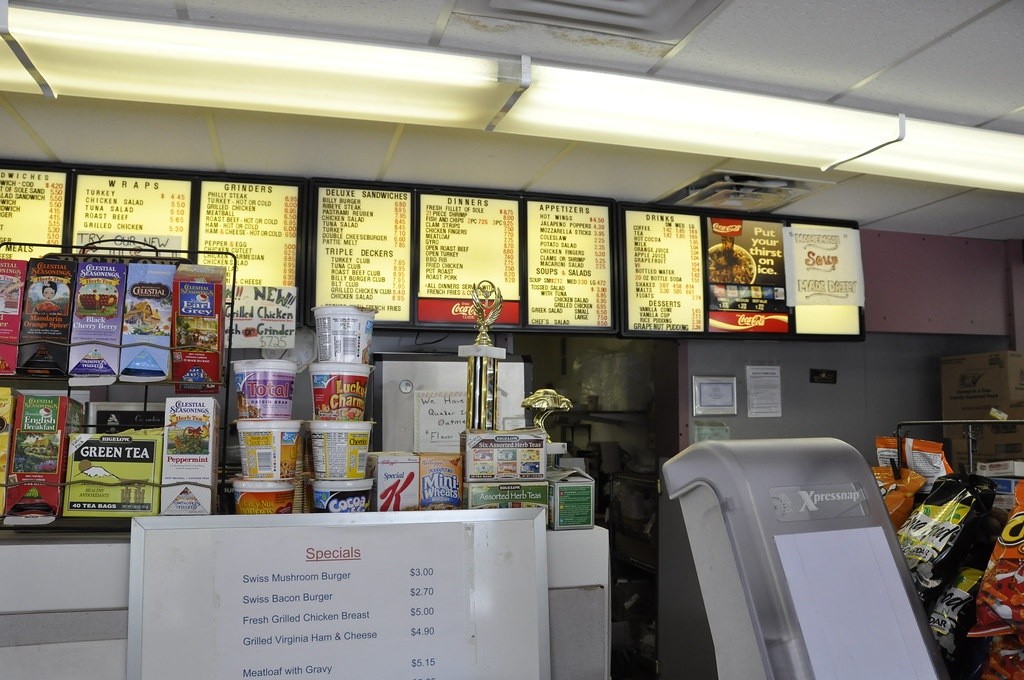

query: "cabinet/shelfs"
left=0, top=239, right=238, bottom=531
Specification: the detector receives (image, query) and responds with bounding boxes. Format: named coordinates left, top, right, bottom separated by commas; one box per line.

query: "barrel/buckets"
left=231, top=305, right=375, bottom=515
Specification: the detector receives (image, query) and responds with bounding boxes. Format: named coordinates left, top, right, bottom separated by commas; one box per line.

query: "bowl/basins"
left=708, top=243, right=757, bottom=284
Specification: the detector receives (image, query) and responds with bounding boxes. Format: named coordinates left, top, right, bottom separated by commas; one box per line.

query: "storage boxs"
left=940, top=351, right=1024, bottom=518
left=367, top=431, right=595, bottom=531
left=0, top=258, right=226, bottom=519
